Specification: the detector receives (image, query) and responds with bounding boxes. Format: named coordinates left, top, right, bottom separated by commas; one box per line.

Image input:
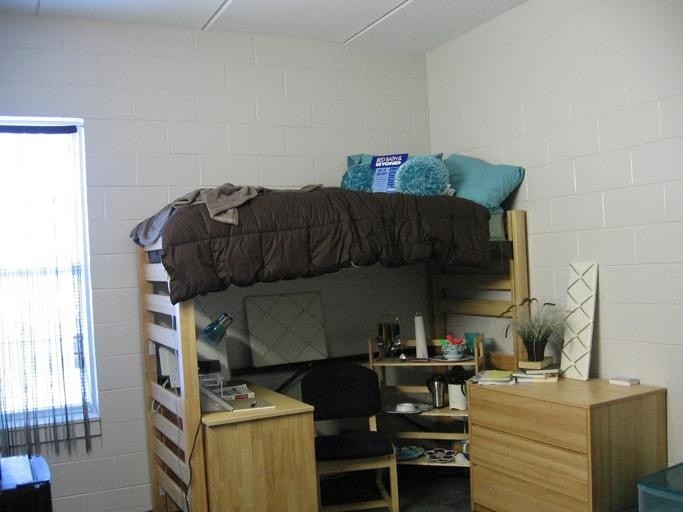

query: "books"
left=511, top=371, right=553, bottom=378
left=368, top=153, right=408, bottom=196
left=480, top=370, right=513, bottom=380
left=608, top=376, right=639, bottom=386
left=210, top=383, right=255, bottom=402
left=515, top=377, right=558, bottom=383
left=477, top=378, right=515, bottom=385
left=517, top=356, right=552, bottom=369
left=521, top=364, right=559, bottom=374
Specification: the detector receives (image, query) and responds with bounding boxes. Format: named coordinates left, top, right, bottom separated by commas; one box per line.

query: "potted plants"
left=497, top=298, right=557, bottom=361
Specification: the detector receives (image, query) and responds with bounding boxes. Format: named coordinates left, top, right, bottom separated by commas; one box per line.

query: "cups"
left=427, top=376, right=444, bottom=408
left=443, top=332, right=480, bottom=360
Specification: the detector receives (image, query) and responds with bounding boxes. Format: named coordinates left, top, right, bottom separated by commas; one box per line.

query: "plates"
left=395, top=445, right=458, bottom=463
left=385, top=408, right=422, bottom=414
left=431, top=355, right=473, bottom=362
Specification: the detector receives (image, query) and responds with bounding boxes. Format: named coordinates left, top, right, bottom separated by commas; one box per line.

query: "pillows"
left=341, top=153, right=444, bottom=194
left=442, top=155, right=526, bottom=206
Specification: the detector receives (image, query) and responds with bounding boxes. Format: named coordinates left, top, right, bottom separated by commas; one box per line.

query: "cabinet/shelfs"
left=466, top=376, right=667, bottom=512
left=161, top=380, right=320, bottom=512
left=368, top=336, right=485, bottom=468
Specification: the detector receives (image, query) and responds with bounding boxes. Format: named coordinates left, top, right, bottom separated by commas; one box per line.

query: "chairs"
left=301, top=365, right=400, bottom=511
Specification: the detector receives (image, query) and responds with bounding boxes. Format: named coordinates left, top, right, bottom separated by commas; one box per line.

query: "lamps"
left=194, top=297, right=234, bottom=344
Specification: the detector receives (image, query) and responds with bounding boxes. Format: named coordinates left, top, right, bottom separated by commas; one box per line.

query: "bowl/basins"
left=395, top=403, right=415, bottom=411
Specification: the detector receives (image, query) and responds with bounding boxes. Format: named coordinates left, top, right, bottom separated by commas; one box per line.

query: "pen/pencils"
left=251, top=400, right=256, bottom=407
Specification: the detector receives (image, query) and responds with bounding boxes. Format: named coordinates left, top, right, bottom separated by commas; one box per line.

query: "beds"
left=127, top=189, right=529, bottom=512
